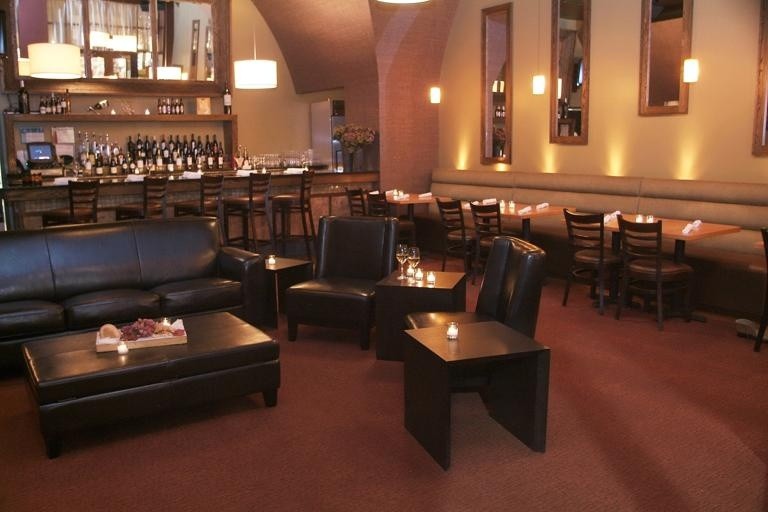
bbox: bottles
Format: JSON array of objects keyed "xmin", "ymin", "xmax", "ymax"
[
  {"xmin": 75, "ymin": 129, "xmax": 225, "ymax": 177},
  {"xmin": 232, "ymin": 144, "xmax": 248, "ymax": 170},
  {"xmin": 495, "ymin": 105, "xmax": 505, "ymax": 116},
  {"xmin": 250, "ymin": 152, "xmax": 306, "ymax": 169},
  {"xmin": 39, "ymin": 89, "xmax": 71, "ymax": 114},
  {"xmin": 157, "ymin": 98, "xmax": 184, "ymax": 114},
  {"xmin": 223, "ymin": 81, "xmax": 232, "ymax": 113},
  {"xmin": 557, "ymin": 96, "xmax": 568, "ymax": 121},
  {"xmin": 87, "ymin": 99, "xmax": 109, "ymax": 112},
  {"xmin": 17, "ymin": 78, "xmax": 30, "ymax": 114},
  {"xmin": 22, "ymin": 172, "xmax": 44, "ymax": 186}
]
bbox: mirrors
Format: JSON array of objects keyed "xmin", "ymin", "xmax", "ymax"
[
  {"xmin": 478, "ymin": 1, "xmax": 513, "ymax": 168},
  {"xmin": 635, "ymin": 0, "xmax": 696, "ymax": 119},
  {"xmin": 7, "ymin": 0, "xmax": 232, "ymax": 98},
  {"xmin": 548, "ymin": 0, "xmax": 591, "ymax": 149}
]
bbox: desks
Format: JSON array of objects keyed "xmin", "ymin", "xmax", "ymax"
[{"xmin": 393, "ymin": 314, "xmax": 560, "ymax": 474}]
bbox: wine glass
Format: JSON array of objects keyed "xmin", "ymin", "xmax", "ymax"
[{"xmin": 394, "ymin": 243, "xmax": 422, "ymax": 283}]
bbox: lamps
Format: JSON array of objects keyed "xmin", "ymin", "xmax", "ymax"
[
  {"xmin": 228, "ymin": 29, "xmax": 279, "ymax": 90},
  {"xmin": 681, "ymin": 57, "xmax": 700, "ymax": 84},
  {"xmin": 428, "ymin": 85, "xmax": 442, "ymax": 105},
  {"xmin": 16, "ymin": 28, "xmax": 87, "ymax": 82},
  {"xmin": 530, "ymin": 73, "xmax": 547, "ymax": 96}
]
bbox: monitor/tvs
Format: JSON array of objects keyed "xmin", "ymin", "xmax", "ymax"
[{"xmin": 27, "ymin": 142, "xmax": 55, "ymax": 163}]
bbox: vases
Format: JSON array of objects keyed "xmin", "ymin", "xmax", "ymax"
[{"xmin": 340, "ymin": 145, "xmax": 366, "ymax": 175}]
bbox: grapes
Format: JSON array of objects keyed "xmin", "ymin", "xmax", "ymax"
[{"xmin": 120, "ymin": 318, "xmax": 156, "ymax": 341}]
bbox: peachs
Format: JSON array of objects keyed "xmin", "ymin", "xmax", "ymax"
[{"xmin": 100, "ymin": 324, "xmax": 119, "ymax": 338}]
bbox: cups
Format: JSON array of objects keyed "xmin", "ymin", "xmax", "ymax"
[
  {"xmin": 413, "ymin": 267, "xmax": 424, "ymax": 280},
  {"xmin": 447, "ymin": 321, "xmax": 458, "ymax": 341},
  {"xmin": 509, "ymin": 200, "xmax": 516, "ymax": 209},
  {"xmin": 499, "ymin": 199, "xmax": 505, "ymax": 207},
  {"xmin": 392, "ymin": 189, "xmax": 403, "ymax": 197},
  {"xmin": 647, "ymin": 213, "xmax": 652, "ymax": 224},
  {"xmin": 162, "ymin": 317, "xmax": 171, "ymax": 325},
  {"xmin": 426, "ymin": 270, "xmax": 437, "ymax": 283},
  {"xmin": 636, "ymin": 214, "xmax": 642, "ymax": 223},
  {"xmin": 268, "ymin": 254, "xmax": 275, "ymax": 265},
  {"xmin": 117, "ymin": 339, "xmax": 129, "ymax": 353}
]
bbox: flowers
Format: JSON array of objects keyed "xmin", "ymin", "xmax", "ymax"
[{"xmin": 328, "ymin": 117, "xmax": 380, "ymax": 158}]
